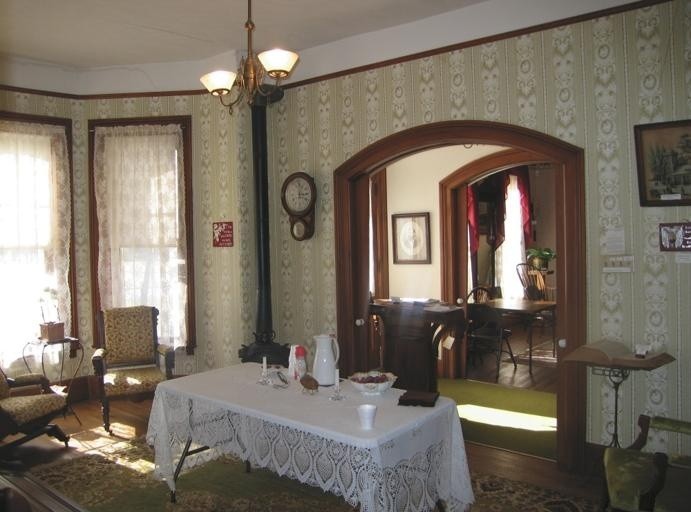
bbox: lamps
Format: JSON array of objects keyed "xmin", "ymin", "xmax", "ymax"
[{"xmin": 200, "ymin": 0, "xmax": 300, "ymax": 115}]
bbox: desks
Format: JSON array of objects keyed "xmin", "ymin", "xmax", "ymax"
[{"xmin": 356, "ymin": 299, "xmax": 463, "ymax": 380}]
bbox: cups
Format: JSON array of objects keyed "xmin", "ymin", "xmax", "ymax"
[{"xmin": 356, "ymin": 404, "xmax": 378, "ymax": 431}]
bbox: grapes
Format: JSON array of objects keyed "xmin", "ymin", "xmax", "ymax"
[{"xmin": 357, "ymin": 375, "xmax": 386, "ymax": 384}]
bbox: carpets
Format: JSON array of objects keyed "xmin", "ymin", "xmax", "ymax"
[{"xmin": 29, "ymin": 433, "xmax": 604, "ymax": 512}]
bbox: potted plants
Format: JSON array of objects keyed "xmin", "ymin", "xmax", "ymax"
[{"xmin": 526, "ymin": 246, "xmax": 556, "ymax": 271}]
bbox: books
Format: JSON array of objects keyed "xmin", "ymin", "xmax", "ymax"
[{"xmin": 561, "ymin": 336, "xmax": 679, "ymax": 369}]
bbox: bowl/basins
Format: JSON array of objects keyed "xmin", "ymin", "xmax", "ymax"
[{"xmin": 347, "ymin": 369, "xmax": 400, "ymax": 396}]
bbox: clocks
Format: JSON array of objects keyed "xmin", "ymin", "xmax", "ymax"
[{"xmin": 280, "ymin": 172, "xmax": 317, "ymax": 241}]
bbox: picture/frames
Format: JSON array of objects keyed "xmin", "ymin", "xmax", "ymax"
[
  {"xmin": 391, "ymin": 212, "xmax": 431, "ymax": 264},
  {"xmin": 634, "ymin": 118, "xmax": 691, "ymax": 252}
]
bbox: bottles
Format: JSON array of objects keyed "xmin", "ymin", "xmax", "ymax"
[{"xmin": 288, "ymin": 344, "xmax": 307, "ymax": 384}]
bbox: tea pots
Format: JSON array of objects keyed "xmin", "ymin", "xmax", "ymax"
[{"xmin": 312, "ymin": 332, "xmax": 342, "ymax": 386}]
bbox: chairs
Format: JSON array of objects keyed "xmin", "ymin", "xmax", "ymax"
[
  {"xmin": 0, "ymin": 305, "xmax": 174, "ymax": 465},
  {"xmin": 461, "ymin": 263, "xmax": 557, "ymax": 379}
]
